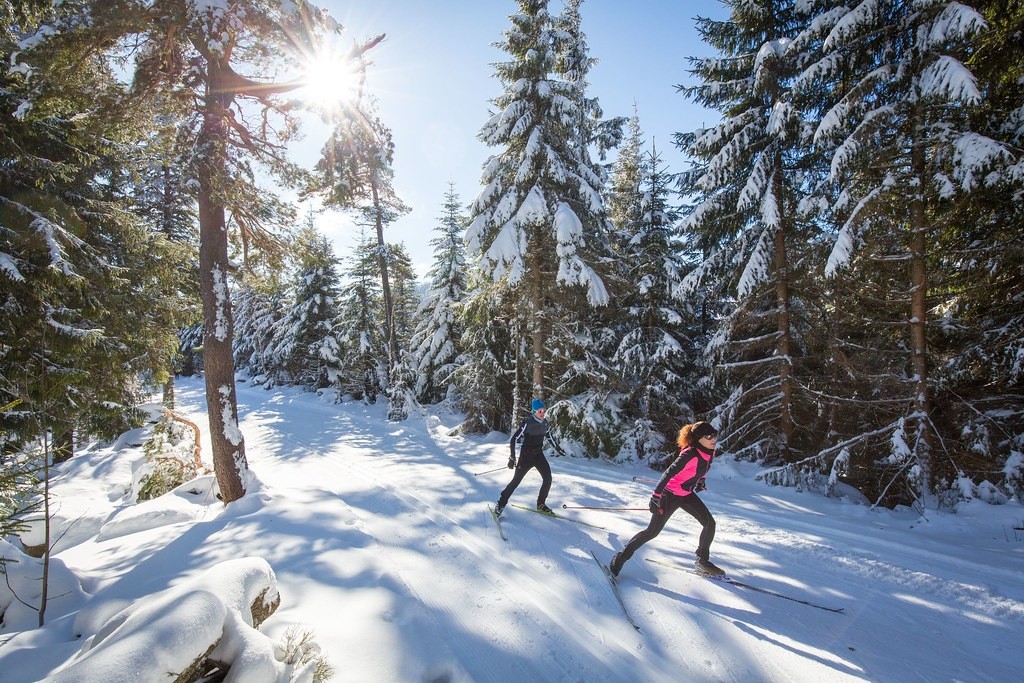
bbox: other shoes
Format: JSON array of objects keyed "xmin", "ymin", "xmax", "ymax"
[
  {"xmin": 610, "ymin": 552, "xmax": 623, "ymax": 576},
  {"xmin": 537, "ymin": 504, "xmax": 551, "ymax": 513},
  {"xmin": 697, "ymin": 559, "xmax": 725, "ymax": 576},
  {"xmin": 495, "ymin": 501, "xmax": 504, "ymax": 515}
]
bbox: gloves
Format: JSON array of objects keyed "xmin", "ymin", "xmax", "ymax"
[
  {"xmin": 556, "ymin": 448, "xmax": 565, "ymax": 455},
  {"xmin": 649, "ymin": 495, "xmax": 660, "ymax": 513},
  {"xmin": 508, "ymin": 459, "xmax": 515, "ymax": 469},
  {"xmin": 696, "ymin": 478, "xmax": 705, "ymax": 493}
]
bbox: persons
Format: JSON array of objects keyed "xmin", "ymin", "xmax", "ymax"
[
  {"xmin": 494, "ymin": 399, "xmax": 566, "ymax": 517},
  {"xmin": 610, "ymin": 421, "xmax": 725, "ymax": 579}
]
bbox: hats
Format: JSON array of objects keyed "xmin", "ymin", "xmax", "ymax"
[{"xmin": 532, "ymin": 400, "xmax": 544, "ymax": 414}]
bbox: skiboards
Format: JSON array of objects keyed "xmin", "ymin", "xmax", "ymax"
[
  {"xmin": 589, "ymin": 550, "xmax": 844, "ymax": 629},
  {"xmin": 487, "ymin": 504, "xmax": 605, "ymax": 542}
]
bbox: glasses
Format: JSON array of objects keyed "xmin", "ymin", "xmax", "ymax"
[
  {"xmin": 533, "ymin": 409, "xmax": 546, "ymax": 414},
  {"xmin": 703, "ymin": 430, "xmax": 717, "ymax": 440}
]
